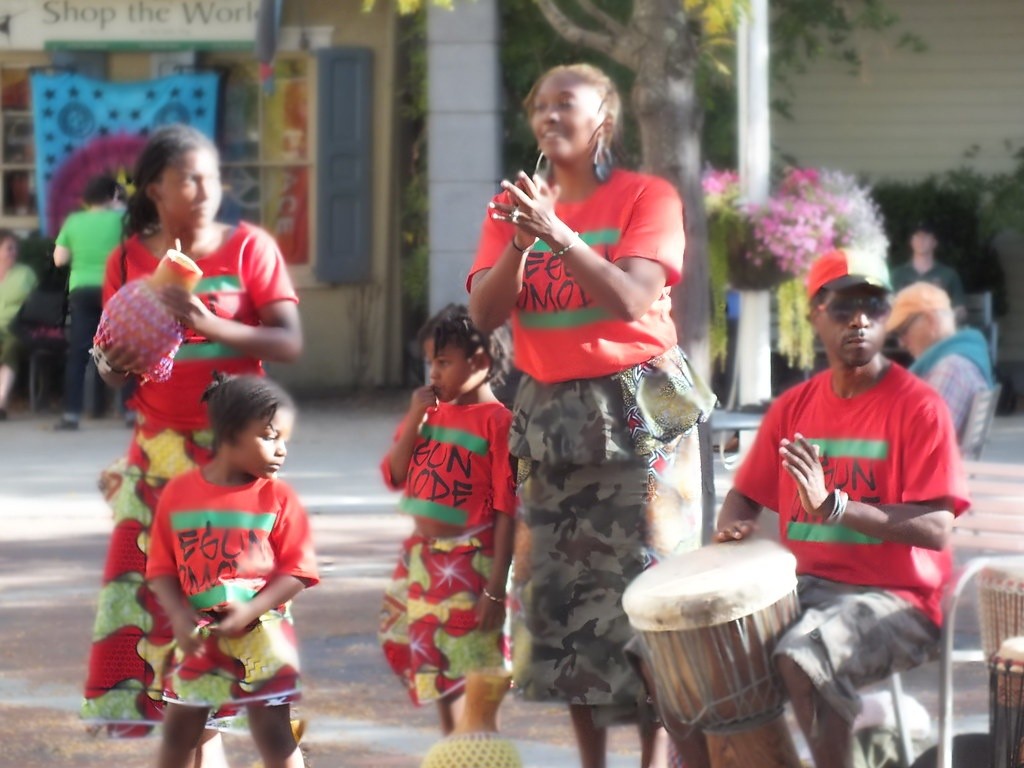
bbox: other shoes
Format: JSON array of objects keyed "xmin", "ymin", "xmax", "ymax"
[
  {"xmin": 53, "ymin": 417, "xmax": 76, "ymax": 428},
  {"xmin": 124, "ymin": 419, "xmax": 135, "ymax": 426}
]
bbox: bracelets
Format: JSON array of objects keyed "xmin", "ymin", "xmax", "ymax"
[
  {"xmin": 481, "ymin": 588, "xmax": 506, "ymax": 602},
  {"xmin": 548, "ymin": 231, "xmax": 579, "ymax": 256},
  {"xmin": 512, "ymin": 235, "xmax": 524, "ymax": 252},
  {"xmin": 822, "ymin": 486, "xmax": 850, "ymax": 526}
]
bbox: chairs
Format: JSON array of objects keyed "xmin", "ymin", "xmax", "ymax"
[
  {"xmin": 964, "ymin": 290, "xmax": 999, "ymax": 368},
  {"xmin": 7, "ymin": 289, "xmax": 69, "ymax": 411},
  {"xmin": 953, "ymin": 384, "xmax": 1004, "ymax": 451}
]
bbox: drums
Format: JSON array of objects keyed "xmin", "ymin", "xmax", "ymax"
[
  {"xmin": 618, "ymin": 541, "xmax": 805, "ymax": 768},
  {"xmin": 995, "ymin": 637, "xmax": 1023, "ymax": 768},
  {"xmin": 974, "ymin": 559, "xmax": 1024, "ymax": 768}
]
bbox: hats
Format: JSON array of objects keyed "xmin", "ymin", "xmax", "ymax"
[
  {"xmin": 809, "ymin": 249, "xmax": 890, "ymax": 305},
  {"xmin": 907, "ymin": 219, "xmax": 941, "ymax": 241}
]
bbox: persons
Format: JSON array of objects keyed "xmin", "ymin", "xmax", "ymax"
[
  {"xmin": 373, "ymin": 300, "xmax": 517, "ymax": 732},
  {"xmin": 884, "ymin": 282, "xmax": 994, "ymax": 445},
  {"xmin": 145, "ymin": 369, "xmax": 323, "ymax": 768},
  {"xmin": 650, "ymin": 246, "xmax": 972, "ymax": 767},
  {"xmin": 461, "ymin": 60, "xmax": 704, "ymax": 768},
  {"xmin": 72, "ymin": 122, "xmax": 303, "ymax": 768},
  {"xmin": 45, "ymin": 177, "xmax": 140, "ymax": 431},
  {"xmin": 0, "ymin": 227, "xmax": 41, "ymax": 422},
  {"xmin": 889, "ymin": 218, "xmax": 968, "ymax": 326}
]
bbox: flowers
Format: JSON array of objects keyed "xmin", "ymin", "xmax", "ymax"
[{"xmin": 702, "ymin": 162, "xmax": 902, "ymax": 370}]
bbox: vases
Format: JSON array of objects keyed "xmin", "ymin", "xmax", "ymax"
[{"xmin": 727, "ymin": 252, "xmax": 788, "ymax": 294}]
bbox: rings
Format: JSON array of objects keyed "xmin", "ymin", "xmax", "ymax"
[{"xmin": 512, "ymin": 207, "xmax": 521, "ymax": 219}]
